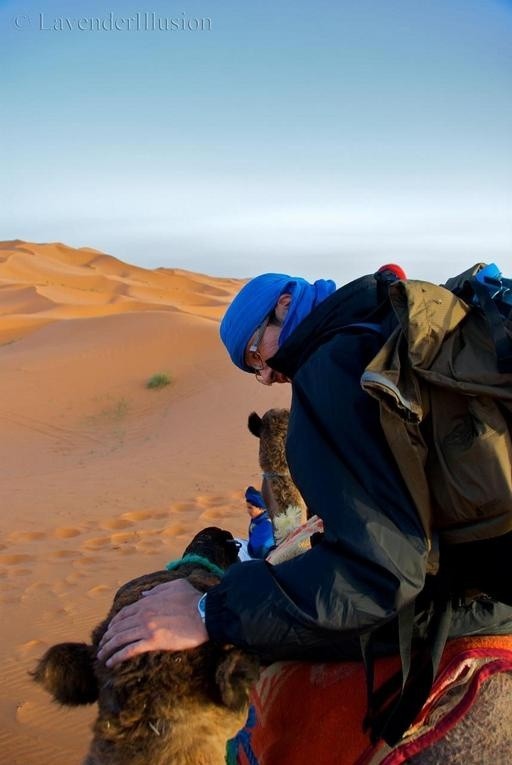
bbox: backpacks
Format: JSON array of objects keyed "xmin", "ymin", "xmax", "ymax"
[{"xmin": 360, "ymin": 260, "xmax": 511, "ymax": 578}]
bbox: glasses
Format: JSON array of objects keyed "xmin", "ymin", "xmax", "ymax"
[{"xmin": 247, "ymin": 314, "xmax": 272, "ymax": 372}]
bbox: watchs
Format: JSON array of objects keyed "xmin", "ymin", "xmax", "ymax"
[{"xmin": 197, "ymin": 590, "xmax": 208, "ymax": 623}]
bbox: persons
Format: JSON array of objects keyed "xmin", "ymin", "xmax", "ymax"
[
  {"xmin": 95, "ymin": 256, "xmax": 512, "ymax": 765},
  {"xmin": 241, "ymin": 485, "xmax": 280, "ymax": 565}
]
